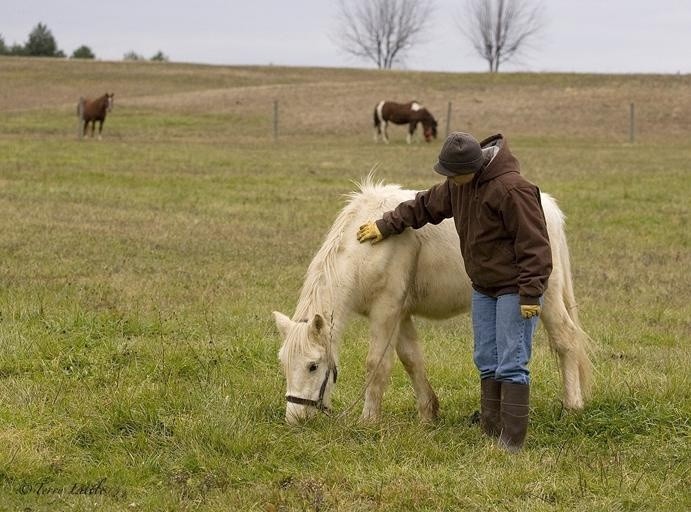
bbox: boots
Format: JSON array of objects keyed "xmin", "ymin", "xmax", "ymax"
[{"xmin": 480, "ymin": 379, "xmax": 530, "ymax": 446}]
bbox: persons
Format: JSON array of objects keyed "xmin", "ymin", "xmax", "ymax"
[{"xmin": 356, "ymin": 133, "xmax": 553, "ymax": 450}]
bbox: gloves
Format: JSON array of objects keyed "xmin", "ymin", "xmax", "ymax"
[
  {"xmin": 357, "ymin": 222, "xmax": 383, "ymax": 244},
  {"xmin": 521, "ymin": 304, "xmax": 540, "ymax": 319}
]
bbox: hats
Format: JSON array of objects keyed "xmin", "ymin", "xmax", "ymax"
[{"xmin": 434, "ymin": 132, "xmax": 485, "ymax": 176}]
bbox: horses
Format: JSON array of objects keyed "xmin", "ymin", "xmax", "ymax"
[
  {"xmin": 374, "ymin": 101, "xmax": 438, "ymax": 145},
  {"xmin": 273, "ymin": 162, "xmax": 605, "ymax": 426},
  {"xmin": 77, "ymin": 92, "xmax": 114, "ymax": 141}
]
